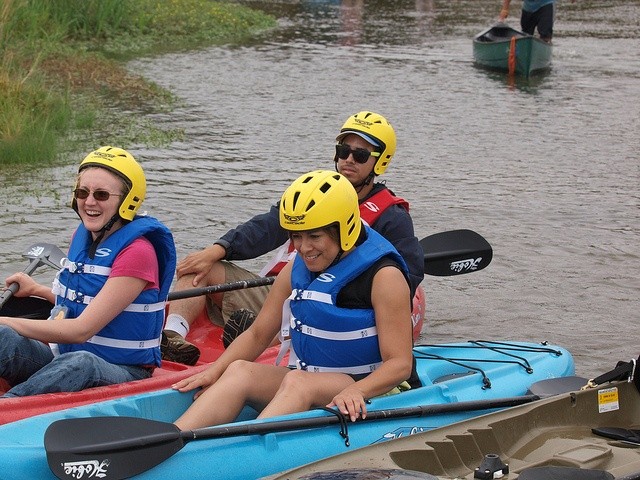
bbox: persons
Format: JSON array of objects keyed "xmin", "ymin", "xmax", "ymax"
[
  {"xmin": 0, "ymin": 146, "xmax": 175, "ymax": 400},
  {"xmin": 172, "ymin": 170, "xmax": 413, "ymax": 432},
  {"xmin": 161, "ymin": 111, "xmax": 425, "ymax": 365},
  {"xmin": 501, "ymin": 1, "xmax": 554, "ymax": 40}
]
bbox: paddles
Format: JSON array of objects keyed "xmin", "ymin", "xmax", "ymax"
[
  {"xmin": 42, "ymin": 374, "xmax": 592, "ymax": 479},
  {"xmin": 0, "ymin": 241, "xmax": 68, "ymax": 311},
  {"xmin": 164, "ymin": 228, "xmax": 493, "ymax": 303}
]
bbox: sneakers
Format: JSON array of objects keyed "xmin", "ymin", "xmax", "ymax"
[
  {"xmin": 158, "ymin": 329, "xmax": 199, "ymax": 366},
  {"xmin": 224, "ymin": 308, "xmax": 257, "ymax": 348}
]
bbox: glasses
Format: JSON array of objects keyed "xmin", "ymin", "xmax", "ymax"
[
  {"xmin": 73, "ymin": 188, "xmax": 124, "ymax": 202},
  {"xmin": 339, "ymin": 143, "xmax": 380, "ymax": 162}
]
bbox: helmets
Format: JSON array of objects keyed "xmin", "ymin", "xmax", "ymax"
[
  {"xmin": 277, "ymin": 170, "xmax": 361, "ymax": 273},
  {"xmin": 72, "ymin": 146, "xmax": 147, "ymax": 261},
  {"xmin": 333, "ymin": 110, "xmax": 397, "ymax": 193}
]
